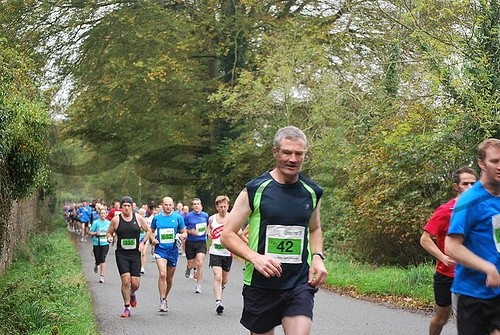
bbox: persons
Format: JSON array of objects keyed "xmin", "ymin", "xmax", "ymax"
[
  {"xmin": 183, "ymin": 198, "xmax": 209, "ymax": 293},
  {"xmin": 420, "ymin": 167, "xmax": 478, "ymax": 335},
  {"xmin": 107, "ymin": 196, "xmax": 151, "ymax": 318},
  {"xmin": 64, "ymin": 199, "xmax": 199, "ymax": 280},
  {"xmin": 221, "ymin": 126, "xmax": 328, "ymax": 335},
  {"xmin": 207, "ymin": 195, "xmax": 249, "ymax": 313},
  {"xmin": 444, "ymin": 139, "xmax": 500, "ymax": 335},
  {"xmin": 88, "ymin": 209, "xmax": 111, "ymax": 284},
  {"xmin": 149, "ymin": 197, "xmax": 188, "ymax": 311}
]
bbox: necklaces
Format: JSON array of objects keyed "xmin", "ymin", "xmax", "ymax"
[{"xmin": 142, "ymin": 239, "xmax": 146, "ymax": 245}]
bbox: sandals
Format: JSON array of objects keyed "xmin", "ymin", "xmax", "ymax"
[{"xmin": 94, "ymin": 264, "xmax": 100, "ymax": 273}]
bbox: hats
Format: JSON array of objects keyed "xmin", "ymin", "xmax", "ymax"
[{"xmin": 120, "ymin": 196, "xmax": 133, "ymax": 208}]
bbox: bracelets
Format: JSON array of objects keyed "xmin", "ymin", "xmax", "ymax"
[
  {"xmin": 95, "ymin": 232, "xmax": 97, "ymax": 235},
  {"xmin": 312, "ymin": 252, "xmax": 325, "ymax": 260}
]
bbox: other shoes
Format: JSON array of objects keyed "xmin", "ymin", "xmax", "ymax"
[
  {"xmin": 195, "ymin": 286, "xmax": 199, "ymax": 293},
  {"xmin": 99, "ymin": 275, "xmax": 104, "ymax": 283},
  {"xmin": 159, "ymin": 300, "xmax": 169, "ymax": 312},
  {"xmin": 130, "ymin": 295, "xmax": 136, "ymax": 307},
  {"xmin": 216, "ymin": 301, "xmax": 224, "ymax": 314},
  {"xmin": 121, "ymin": 308, "xmax": 130, "ymax": 317},
  {"xmin": 72, "ymin": 228, "xmax": 89, "ymax": 242},
  {"xmin": 185, "ymin": 269, "xmax": 191, "ymax": 278}
]
